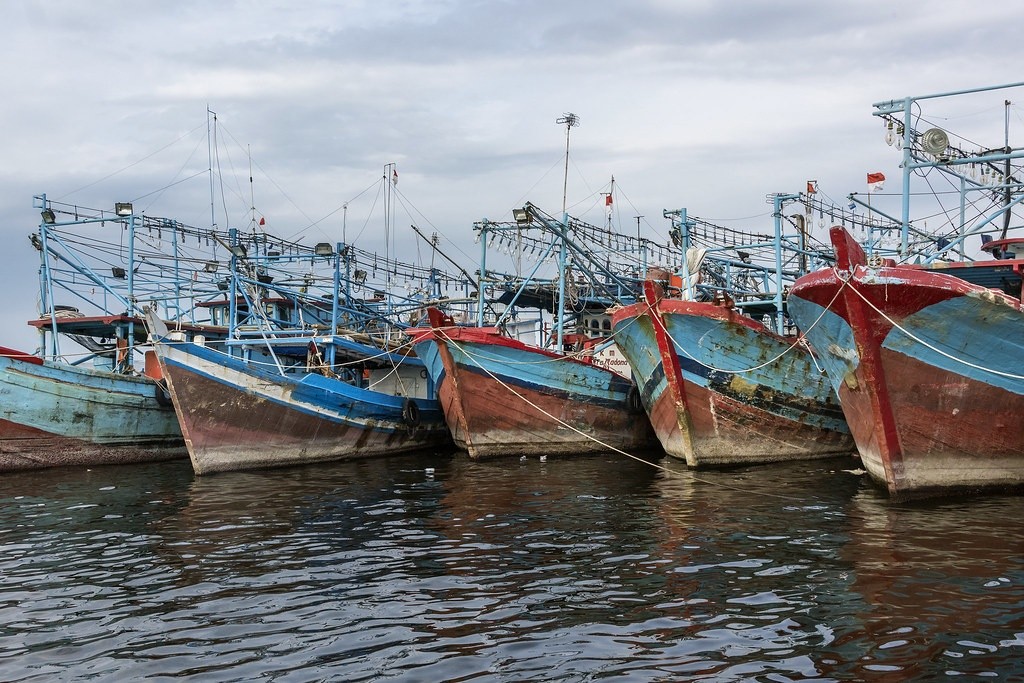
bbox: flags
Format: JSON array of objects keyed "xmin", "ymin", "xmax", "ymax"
[
  {"xmin": 808, "ymin": 183, "xmax": 817, "ymax": 194},
  {"xmin": 259, "ymin": 218, "xmax": 265, "ymax": 225},
  {"xmin": 868, "ymin": 173, "xmax": 885, "ymax": 184},
  {"xmin": 606, "ymin": 196, "xmax": 612, "ymax": 206}
]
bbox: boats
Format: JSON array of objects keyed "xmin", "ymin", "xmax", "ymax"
[{"xmin": 0, "ymin": 80, "xmax": 1024, "ymax": 503}]
